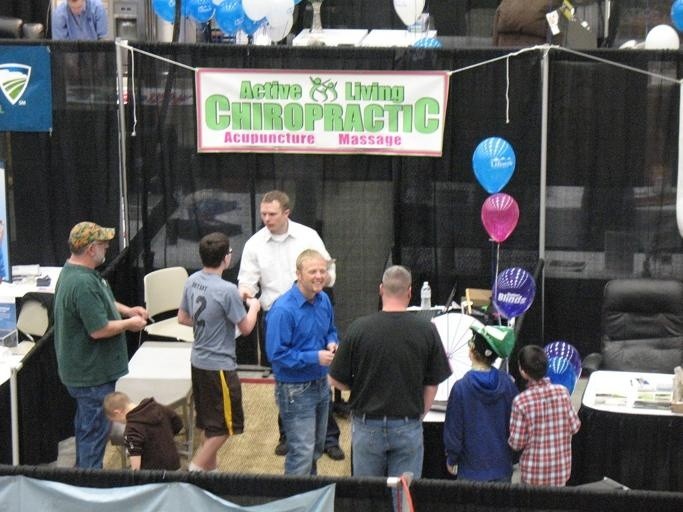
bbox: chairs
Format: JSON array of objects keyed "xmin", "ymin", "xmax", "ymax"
[
  {"xmin": 138, "ymin": 265, "xmax": 191, "ymax": 346},
  {"xmin": 582, "ymin": 277, "xmax": 683, "ymax": 373}
]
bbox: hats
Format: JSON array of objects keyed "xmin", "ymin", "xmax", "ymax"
[
  {"xmin": 469, "ymin": 325, "xmax": 516, "ymax": 361},
  {"xmin": 68, "ymin": 222, "xmax": 116, "ymax": 253}
]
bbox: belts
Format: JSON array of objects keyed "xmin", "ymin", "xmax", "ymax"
[{"xmin": 353, "ymin": 408, "xmax": 417, "ymax": 420}]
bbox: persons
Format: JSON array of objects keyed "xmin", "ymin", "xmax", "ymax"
[
  {"xmin": 53, "ymin": 221, "xmax": 151, "ymax": 470},
  {"xmin": 103, "ymin": 390, "xmax": 184, "ymax": 470},
  {"xmin": 327, "ymin": 265, "xmax": 453, "ymax": 480},
  {"xmin": 490, "ymin": 0, "xmax": 565, "ymax": 46},
  {"xmin": 265, "ymin": 246, "xmax": 343, "ymax": 478},
  {"xmin": 176, "ymin": 230, "xmax": 263, "ymax": 475},
  {"xmin": 234, "ymin": 189, "xmax": 347, "ymax": 462},
  {"xmin": 504, "ymin": 344, "xmax": 582, "ymax": 486},
  {"xmin": 441, "ymin": 323, "xmax": 521, "ymax": 482},
  {"xmin": 51, "ymin": 0, "xmax": 111, "ymax": 39}
]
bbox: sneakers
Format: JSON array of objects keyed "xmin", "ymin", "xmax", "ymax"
[
  {"xmin": 276, "ymin": 441, "xmax": 288, "ymax": 454},
  {"xmin": 325, "ymin": 445, "xmax": 344, "ymax": 459}
]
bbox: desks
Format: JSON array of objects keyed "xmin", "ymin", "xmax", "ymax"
[
  {"xmin": 579, "ymin": 370, "xmax": 682, "ymax": 495},
  {"xmin": 115, "ymin": 339, "xmax": 202, "ymax": 461}
]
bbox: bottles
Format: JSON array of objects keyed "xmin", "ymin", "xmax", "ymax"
[{"xmin": 418, "ymin": 280, "xmax": 433, "ymax": 308}]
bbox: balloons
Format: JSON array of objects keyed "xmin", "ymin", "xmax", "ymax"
[
  {"xmin": 645, "ymin": 0, "xmax": 682, "ymax": 50},
  {"xmin": 480, "ymin": 192, "xmax": 521, "ymax": 242},
  {"xmin": 546, "ymin": 355, "xmax": 578, "ymax": 396},
  {"xmin": 542, "ymin": 339, "xmax": 584, "ymax": 380},
  {"xmin": 491, "ymin": 267, "xmax": 537, "ymax": 319},
  {"xmin": 471, "ymin": 136, "xmax": 516, "ymax": 194},
  {"xmin": 389, "ymin": 0, "xmax": 442, "ymax": 48},
  {"xmin": 150, "ymin": 0, "xmax": 302, "ymax": 43}
]
